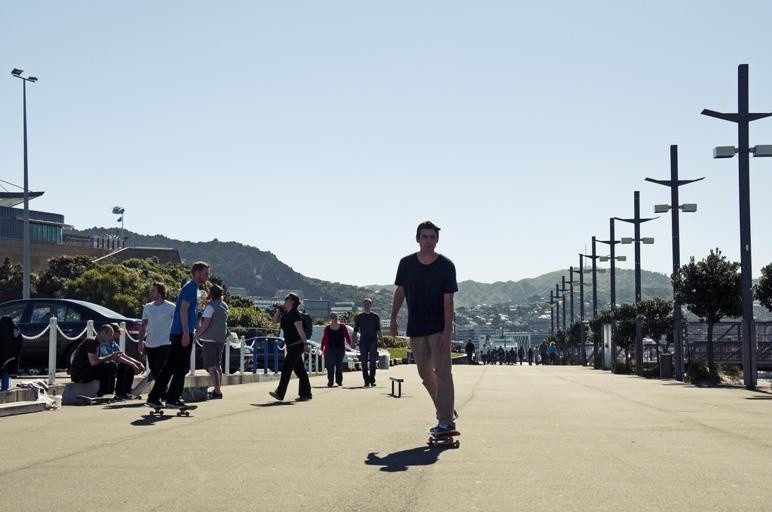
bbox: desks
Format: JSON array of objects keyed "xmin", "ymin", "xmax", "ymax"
[
  {"xmin": 546, "ymin": 189, "xmax": 660, "ymax": 375},
  {"xmin": 644, "ymin": 145, "xmax": 705, "ymax": 382},
  {"xmin": 700, "ymin": 63, "xmax": 771, "ymax": 387},
  {"xmin": 11, "ymin": 68, "xmax": 38, "ymax": 300}
]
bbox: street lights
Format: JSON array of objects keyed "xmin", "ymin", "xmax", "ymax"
[{"xmin": 300, "ymin": 313, "xmax": 312, "ymax": 339}]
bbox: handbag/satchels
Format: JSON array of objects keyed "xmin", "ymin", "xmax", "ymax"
[{"xmin": 303, "ymin": 343, "xmax": 308, "ymax": 346}]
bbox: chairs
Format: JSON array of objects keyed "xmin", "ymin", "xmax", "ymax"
[
  {"xmin": 328, "ymin": 380, "xmax": 342, "ymax": 386},
  {"xmin": 166, "ymin": 401, "xmax": 186, "ymax": 408},
  {"xmin": 453, "ymin": 411, "xmax": 458, "ymax": 419},
  {"xmin": 296, "ymin": 394, "xmax": 313, "ymax": 401},
  {"xmin": 270, "ymin": 392, "xmax": 281, "ymax": 400},
  {"xmin": 147, "ymin": 399, "xmax": 163, "ymax": 407},
  {"xmin": 430, "ymin": 424, "xmax": 455, "ymax": 433},
  {"xmin": 364, "ymin": 383, "xmax": 376, "ymax": 387},
  {"xmin": 209, "ymin": 390, "xmax": 223, "ymax": 399}
]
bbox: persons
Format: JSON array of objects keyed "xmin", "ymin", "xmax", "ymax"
[
  {"xmin": 72, "ymin": 324, "xmax": 124, "ymax": 402},
  {"xmin": 463, "ymin": 339, "xmax": 558, "ymax": 366},
  {"xmin": 351, "ymin": 297, "xmax": 388, "ymax": 389},
  {"xmin": 390, "ymin": 223, "xmax": 459, "ymax": 435},
  {"xmin": 138, "ymin": 281, "xmax": 176, "ymax": 404},
  {"xmin": 198, "ymin": 281, "xmax": 230, "ymax": 397},
  {"xmin": 99, "ymin": 323, "xmax": 146, "ymax": 402},
  {"xmin": 147, "ymin": 261, "xmax": 211, "ymax": 408},
  {"xmin": 271, "ymin": 291, "xmax": 314, "ymax": 401},
  {"xmin": 319, "ymin": 313, "xmax": 356, "ymax": 388}
]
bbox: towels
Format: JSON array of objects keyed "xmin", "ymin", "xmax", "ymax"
[{"xmin": 659, "ymin": 354, "xmax": 673, "ymax": 379}]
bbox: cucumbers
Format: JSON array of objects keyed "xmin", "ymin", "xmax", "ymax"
[
  {"xmin": 429, "ymin": 429, "xmax": 461, "ymax": 447},
  {"xmin": 129, "ymin": 371, "xmax": 153, "ymax": 399},
  {"xmin": 144, "ymin": 404, "xmax": 197, "ymax": 417},
  {"xmin": 75, "ymin": 393, "xmax": 117, "ymax": 406}
]
